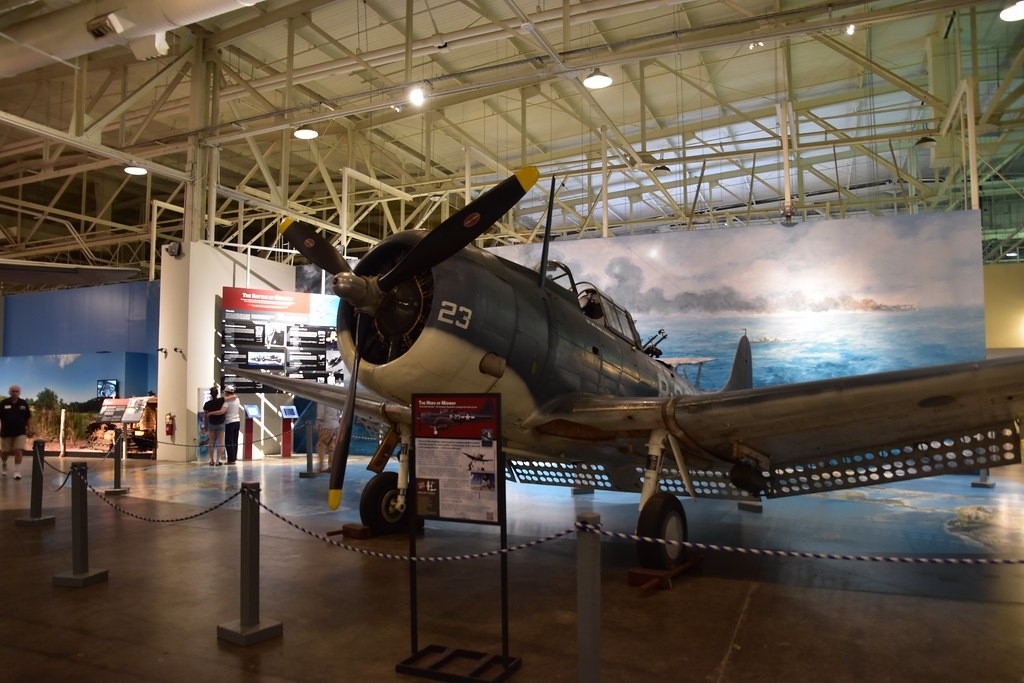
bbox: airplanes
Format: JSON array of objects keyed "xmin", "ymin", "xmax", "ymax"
[{"xmin": 221, "ymin": 163, "xmax": 1024, "ymax": 569}]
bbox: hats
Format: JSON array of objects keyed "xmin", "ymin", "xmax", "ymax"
[
  {"xmin": 210, "ymin": 387, "xmax": 218, "ymax": 394},
  {"xmin": 224, "ymin": 385, "xmax": 235, "ymax": 393}
]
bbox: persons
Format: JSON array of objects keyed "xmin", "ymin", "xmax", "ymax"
[
  {"xmin": 0, "ymin": 385, "xmax": 33, "ymax": 480},
  {"xmin": 202, "ymin": 385, "xmax": 240, "ymax": 466},
  {"xmin": 335, "ymin": 377, "xmax": 344, "ymax": 387},
  {"xmin": 316, "ymin": 402, "xmax": 340, "ymax": 472}
]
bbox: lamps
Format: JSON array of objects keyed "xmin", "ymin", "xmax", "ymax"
[
  {"xmin": 583, "ymin": 68, "xmax": 613, "ymax": 89},
  {"xmin": 124, "ymin": 167, "xmax": 147, "ymax": 176},
  {"xmin": 1000, "ymin": 0, "xmax": 1024, "ymax": 23},
  {"xmin": 293, "ymin": 123, "xmax": 320, "ymax": 139}
]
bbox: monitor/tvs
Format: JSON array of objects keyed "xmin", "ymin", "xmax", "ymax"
[{"xmin": 96, "ymin": 379, "xmax": 118, "ymax": 398}]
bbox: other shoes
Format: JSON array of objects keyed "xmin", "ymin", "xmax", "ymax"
[
  {"xmin": 3, "ymin": 463, "xmax": 7, "ymax": 475},
  {"xmin": 225, "ymin": 460, "xmax": 236, "ymax": 464},
  {"xmin": 324, "ymin": 466, "xmax": 332, "ymax": 472},
  {"xmin": 209, "ymin": 459, "xmax": 214, "ymax": 466},
  {"xmin": 216, "ymin": 461, "xmax": 222, "ymax": 466},
  {"xmin": 14, "ymin": 472, "xmax": 21, "ymax": 479}
]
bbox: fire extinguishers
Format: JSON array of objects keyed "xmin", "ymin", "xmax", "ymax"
[{"xmin": 166, "ymin": 413, "xmax": 176, "ymax": 436}]
